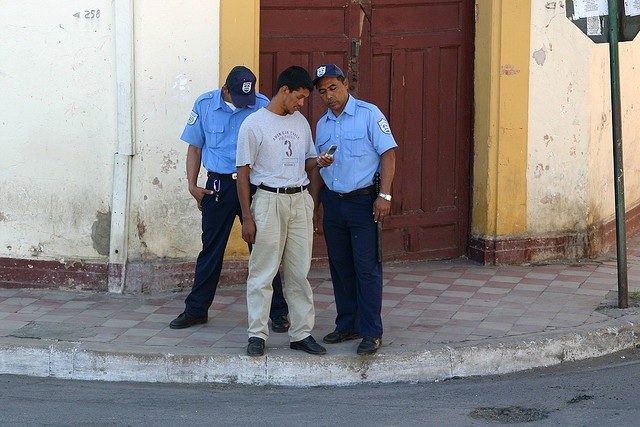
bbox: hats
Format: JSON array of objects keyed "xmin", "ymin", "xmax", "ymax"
[
  {"xmin": 226, "ymin": 65, "xmax": 256, "ymax": 106},
  {"xmin": 311, "ymin": 63, "xmax": 346, "ymax": 84}
]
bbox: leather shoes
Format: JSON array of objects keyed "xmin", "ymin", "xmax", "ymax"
[
  {"xmin": 248, "ymin": 335, "xmax": 265, "ymax": 355},
  {"xmin": 290, "ymin": 335, "xmax": 327, "ymax": 354},
  {"xmin": 323, "ymin": 330, "xmax": 362, "ymax": 343},
  {"xmin": 357, "ymin": 335, "xmax": 382, "ymax": 353},
  {"xmin": 272, "ymin": 314, "xmax": 289, "ymax": 331},
  {"xmin": 170, "ymin": 310, "xmax": 208, "ymax": 329}
]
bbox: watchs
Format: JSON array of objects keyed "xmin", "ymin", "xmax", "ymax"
[{"xmin": 378, "ymin": 192, "xmax": 391, "ymax": 200}]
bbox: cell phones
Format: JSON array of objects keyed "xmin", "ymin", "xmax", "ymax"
[{"xmin": 325, "ymin": 144, "xmax": 337, "ymax": 157}]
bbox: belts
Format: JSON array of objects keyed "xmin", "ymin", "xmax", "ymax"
[
  {"xmin": 205, "ymin": 170, "xmax": 238, "ymax": 179},
  {"xmin": 324, "ymin": 186, "xmax": 377, "ymax": 196},
  {"xmin": 258, "ymin": 182, "xmax": 309, "ymax": 195}
]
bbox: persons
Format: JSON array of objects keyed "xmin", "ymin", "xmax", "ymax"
[
  {"xmin": 235, "ymin": 66, "xmax": 337, "ymax": 355},
  {"xmin": 170, "ymin": 65, "xmax": 289, "ymax": 332},
  {"xmin": 308, "ymin": 64, "xmax": 399, "ymax": 352}
]
